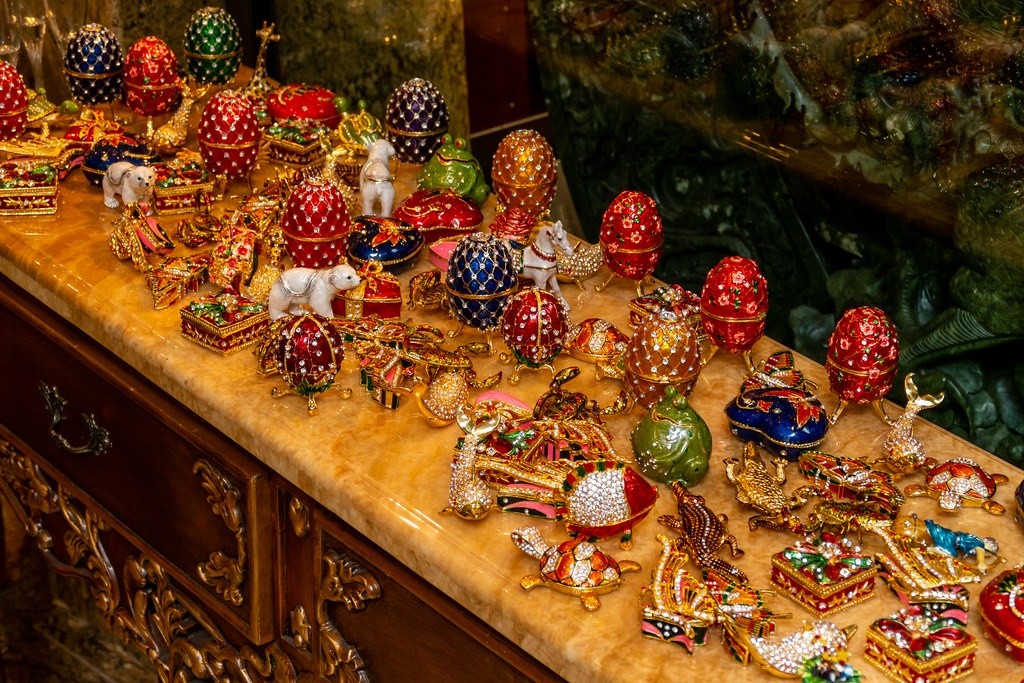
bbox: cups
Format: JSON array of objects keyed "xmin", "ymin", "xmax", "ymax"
[{"xmin": 0, "ymin": 0, "xmax": 21, "ymax": 74}]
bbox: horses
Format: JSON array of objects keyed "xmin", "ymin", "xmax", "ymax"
[{"xmin": 502, "ymin": 219, "xmax": 574, "ymax": 310}]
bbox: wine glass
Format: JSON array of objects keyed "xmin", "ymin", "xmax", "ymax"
[
  {"xmin": 6, "ymin": 0, "xmax": 48, "ymax": 98},
  {"xmin": 43, "ymin": 0, "xmax": 88, "ymax": 58}
]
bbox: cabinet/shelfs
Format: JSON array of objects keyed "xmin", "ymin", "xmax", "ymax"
[{"xmin": 0, "ymin": 64, "xmax": 1024, "ymax": 683}]
